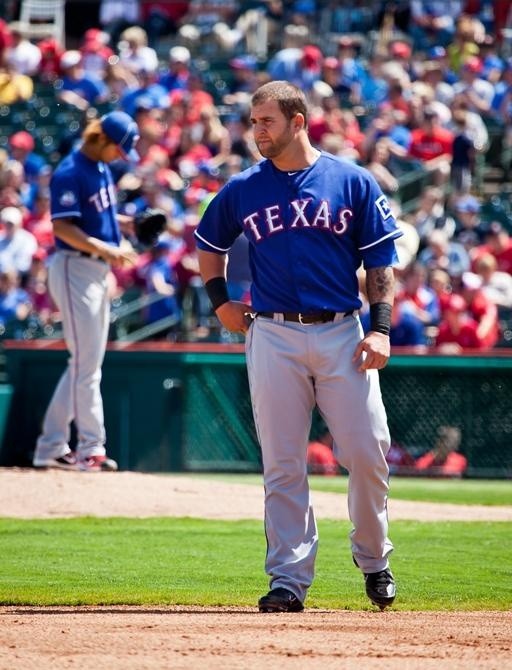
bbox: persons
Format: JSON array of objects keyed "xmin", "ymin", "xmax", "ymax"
[
  {"xmin": 415, "ymin": 423, "xmax": 466, "ymax": 477},
  {"xmin": 306, "ymin": 424, "xmax": 342, "ymax": 477},
  {"xmin": 192, "ymin": 81, "xmax": 401, "ymax": 612},
  {"xmin": 383, "ymin": 441, "xmax": 414, "ymax": 476},
  {"xmin": 1, "ymin": 0, "xmax": 512, "ymax": 355},
  {"xmin": 31, "ymin": 111, "xmax": 142, "ymax": 472}
]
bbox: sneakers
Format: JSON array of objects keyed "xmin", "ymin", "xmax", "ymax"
[
  {"xmin": 352, "ymin": 555, "xmax": 396, "ymax": 607},
  {"xmin": 259, "ymin": 587, "xmax": 304, "ymax": 612},
  {"xmin": 75, "ymin": 450, "xmax": 118, "ymax": 472},
  {"xmin": 33, "ymin": 451, "xmax": 83, "ymax": 470}
]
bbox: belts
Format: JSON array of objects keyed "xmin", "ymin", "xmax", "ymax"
[
  {"xmin": 257, "ymin": 311, "xmax": 353, "ymax": 326},
  {"xmin": 80, "ymin": 251, "xmax": 103, "ymax": 260}
]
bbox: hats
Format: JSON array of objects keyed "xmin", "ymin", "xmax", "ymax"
[{"xmin": 100, "ymin": 110, "xmax": 140, "ymax": 161}]
